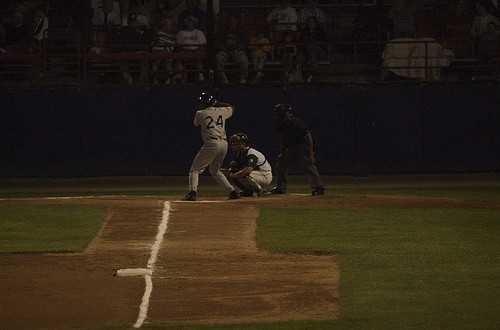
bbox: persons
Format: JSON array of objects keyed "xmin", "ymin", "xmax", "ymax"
[
  {"xmin": 272, "ymin": 102, "xmax": 325, "ymax": 195},
  {"xmin": 227, "ymin": 133, "xmax": 274, "ymax": 197},
  {"xmin": 0, "ymin": 0, "xmax": 500, "ymax": 81},
  {"xmin": 184, "ymin": 90, "xmax": 239, "ymax": 200}
]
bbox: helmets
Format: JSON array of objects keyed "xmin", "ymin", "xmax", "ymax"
[
  {"xmin": 195, "ymin": 89, "xmax": 216, "ymax": 106},
  {"xmin": 274, "ymin": 103, "xmax": 291, "ymax": 113},
  {"xmin": 229, "ymin": 133, "xmax": 248, "ymax": 142}
]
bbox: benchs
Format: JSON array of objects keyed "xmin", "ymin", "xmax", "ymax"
[{"xmin": 0, "ymin": 5, "xmax": 500, "ymax": 82}]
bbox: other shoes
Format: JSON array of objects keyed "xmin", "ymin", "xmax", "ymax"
[
  {"xmin": 312, "ymin": 187, "xmax": 324, "ymax": 196},
  {"xmin": 228, "ymin": 189, "xmax": 240, "ymax": 199},
  {"xmin": 183, "ymin": 190, "xmax": 197, "ymax": 201},
  {"xmin": 239, "ymin": 191, "xmax": 255, "ymax": 197},
  {"xmin": 270, "ymin": 187, "xmax": 287, "ymax": 194}
]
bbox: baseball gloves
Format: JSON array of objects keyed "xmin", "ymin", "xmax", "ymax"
[{"xmin": 223, "ymin": 160, "xmax": 238, "ymax": 170}]
